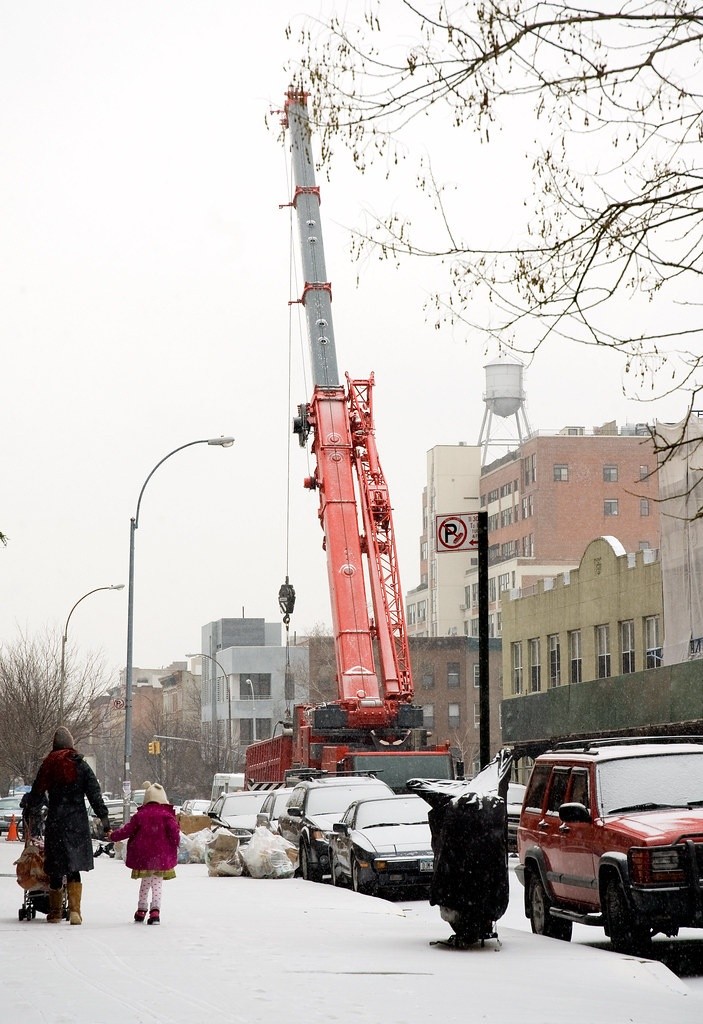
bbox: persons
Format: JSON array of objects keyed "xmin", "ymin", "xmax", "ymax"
[
  {"xmin": 19, "ymin": 781, "xmax": 49, "ymax": 840},
  {"xmin": 107, "ymin": 783, "xmax": 181, "ymax": 925},
  {"xmin": 29, "ymin": 726, "xmax": 112, "ymax": 925}
]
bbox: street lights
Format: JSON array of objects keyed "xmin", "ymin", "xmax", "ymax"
[
  {"xmin": 58, "ymin": 583, "xmax": 126, "ymax": 727},
  {"xmin": 245, "ymin": 679, "xmax": 256, "ymax": 743},
  {"xmin": 123, "ymin": 436, "xmax": 237, "ymax": 827},
  {"xmin": 185, "ymin": 653, "xmax": 231, "ymax": 773}
]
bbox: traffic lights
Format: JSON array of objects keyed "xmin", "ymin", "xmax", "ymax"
[
  {"xmin": 148, "ymin": 742, "xmax": 154, "ymax": 755},
  {"xmin": 155, "ymin": 741, "xmax": 161, "ymax": 755}
]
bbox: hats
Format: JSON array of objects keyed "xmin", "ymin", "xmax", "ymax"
[
  {"xmin": 140, "ymin": 781, "xmax": 169, "ymax": 804},
  {"xmin": 52, "ymin": 726, "xmax": 73, "ymax": 749}
]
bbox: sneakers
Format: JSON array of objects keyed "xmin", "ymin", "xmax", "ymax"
[
  {"xmin": 147, "ymin": 911, "xmax": 160, "ymax": 925},
  {"xmin": 134, "ymin": 911, "xmax": 145, "ymax": 923}
]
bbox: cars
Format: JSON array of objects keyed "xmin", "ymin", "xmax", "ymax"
[
  {"xmin": 0, "ymin": 790, "xmax": 146, "ymax": 841},
  {"xmin": 507, "ymin": 782, "xmax": 528, "ymax": 852},
  {"xmin": 180, "ymin": 800, "xmax": 194, "ymax": 815},
  {"xmin": 256, "ymin": 786, "xmax": 294, "ymax": 834},
  {"xmin": 328, "ymin": 794, "xmax": 433, "ymax": 895},
  {"xmin": 188, "ymin": 799, "xmax": 212, "ymax": 815},
  {"xmin": 206, "ymin": 790, "xmax": 272, "ymax": 845}
]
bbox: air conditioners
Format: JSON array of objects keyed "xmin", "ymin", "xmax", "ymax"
[
  {"xmin": 460, "ymin": 604, "xmax": 467, "ymax": 609},
  {"xmin": 472, "ymin": 601, "xmax": 475, "ymax": 607}
]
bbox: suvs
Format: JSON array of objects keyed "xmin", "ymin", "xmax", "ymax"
[
  {"xmin": 276, "ymin": 775, "xmax": 397, "ymax": 882},
  {"xmin": 514, "ymin": 735, "xmax": 703, "ymax": 960}
]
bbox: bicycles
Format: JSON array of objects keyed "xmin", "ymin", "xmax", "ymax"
[{"xmin": 16, "ymin": 809, "xmax": 46, "ymax": 846}]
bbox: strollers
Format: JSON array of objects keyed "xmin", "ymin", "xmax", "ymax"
[{"xmin": 13, "ymin": 844, "xmax": 71, "ymax": 923}]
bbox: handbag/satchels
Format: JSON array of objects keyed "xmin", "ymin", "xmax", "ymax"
[{"xmin": 13, "ymin": 845, "xmax": 48, "ymax": 891}]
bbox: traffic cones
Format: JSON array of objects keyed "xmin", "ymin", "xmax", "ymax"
[{"xmin": 5, "ymin": 814, "xmax": 18, "ymax": 841}]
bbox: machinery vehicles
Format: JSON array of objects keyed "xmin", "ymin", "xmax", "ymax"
[{"xmin": 244, "ymin": 89, "xmax": 467, "ymax": 791}]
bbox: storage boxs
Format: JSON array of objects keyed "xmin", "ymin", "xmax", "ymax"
[
  {"xmin": 208, "ymin": 850, "xmax": 235, "ymax": 877},
  {"xmin": 208, "ymin": 834, "xmax": 238, "ymax": 852},
  {"xmin": 285, "ymin": 848, "xmax": 298, "ymax": 866}
]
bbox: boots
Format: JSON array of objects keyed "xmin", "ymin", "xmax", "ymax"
[
  {"xmin": 67, "ymin": 882, "xmax": 82, "ymax": 924},
  {"xmin": 46, "ymin": 884, "xmax": 62, "ymax": 922}
]
